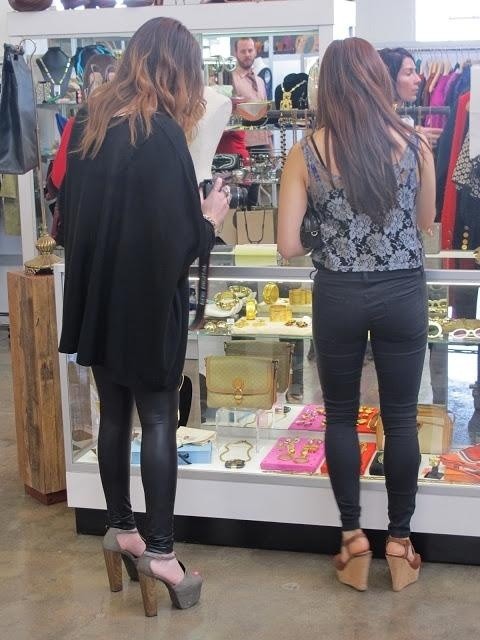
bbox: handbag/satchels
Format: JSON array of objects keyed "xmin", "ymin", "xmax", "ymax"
[
  {"xmin": 369, "ymin": 404, "xmax": 453, "ymax": 455},
  {"xmin": 224, "ymin": 340, "xmax": 295, "ymax": 393},
  {"xmin": 0, "ymin": 43, "xmax": 40, "ymax": 175},
  {"xmin": 198, "ymin": 153, "xmax": 243, "ymax": 208},
  {"xmin": 204, "ymin": 355, "xmax": 279, "ymax": 410},
  {"xmin": 300, "ymin": 209, "xmax": 323, "ymax": 251}
]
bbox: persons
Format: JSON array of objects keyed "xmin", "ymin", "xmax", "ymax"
[
  {"xmin": 378, "ymin": 47, "xmax": 440, "ymax": 147},
  {"xmin": 229, "ymin": 38, "xmax": 271, "ymax": 206},
  {"xmin": 278, "ymin": 36, "xmax": 437, "ymax": 592},
  {"xmin": 55, "ymin": 16, "xmax": 232, "ymax": 618}
]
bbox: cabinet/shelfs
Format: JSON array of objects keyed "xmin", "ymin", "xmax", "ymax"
[
  {"xmin": 9, "ymin": 0, "xmax": 356, "ymax": 265},
  {"xmin": 5, "ymin": 263, "xmax": 68, "ymax": 506}
]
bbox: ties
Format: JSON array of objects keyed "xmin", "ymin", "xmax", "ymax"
[{"xmin": 246, "ymin": 72, "xmax": 258, "ymax": 92}]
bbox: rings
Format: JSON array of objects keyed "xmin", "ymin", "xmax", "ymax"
[
  {"xmin": 226, "ymin": 197, "xmax": 231, "ymax": 205},
  {"xmin": 220, "ymin": 188, "xmax": 229, "ymax": 195}
]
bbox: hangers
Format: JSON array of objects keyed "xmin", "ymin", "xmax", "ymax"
[{"xmin": 408, "ymin": 47, "xmax": 480, "ymax": 99}]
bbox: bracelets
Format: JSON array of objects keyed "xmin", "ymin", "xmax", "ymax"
[
  {"xmin": 204, "ymin": 282, "xmax": 314, "ymax": 334},
  {"xmin": 204, "ymin": 214, "xmax": 217, "ymax": 236}
]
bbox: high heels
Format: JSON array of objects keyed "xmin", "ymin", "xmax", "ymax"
[
  {"xmin": 384, "ymin": 536, "xmax": 422, "ymax": 592},
  {"xmin": 136, "ymin": 549, "xmax": 203, "ymax": 617},
  {"xmin": 332, "ymin": 532, "xmax": 373, "ymax": 591},
  {"xmin": 102, "ymin": 527, "xmax": 147, "ymax": 593}
]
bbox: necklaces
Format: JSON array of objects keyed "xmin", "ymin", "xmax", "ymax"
[
  {"xmin": 39, "ymin": 56, "xmax": 72, "ymax": 97},
  {"xmin": 281, "ymin": 80, "xmax": 306, "ymax": 111},
  {"xmin": 242, "ymin": 210, "xmax": 267, "ymax": 246}
]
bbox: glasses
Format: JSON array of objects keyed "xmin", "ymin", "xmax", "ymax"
[
  {"xmin": 448, "ymin": 328, "xmax": 480, "ymax": 339},
  {"xmin": 427, "ymin": 320, "xmax": 444, "ymax": 339}
]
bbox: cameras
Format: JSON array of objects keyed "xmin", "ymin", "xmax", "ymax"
[{"xmin": 199, "ymin": 179, "xmax": 249, "ymax": 209}]
